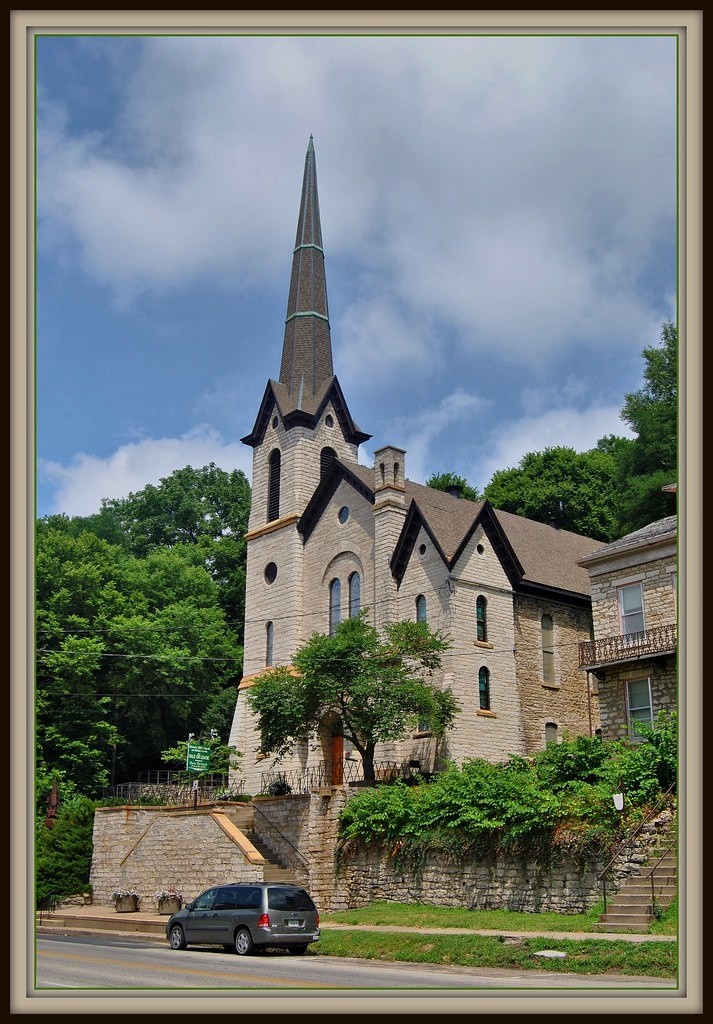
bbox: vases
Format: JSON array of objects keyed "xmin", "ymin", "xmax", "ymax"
[
  {"xmin": 116, "ymin": 896, "xmax": 137, "ymax": 913},
  {"xmin": 158, "ymin": 899, "xmax": 181, "ymax": 915}
]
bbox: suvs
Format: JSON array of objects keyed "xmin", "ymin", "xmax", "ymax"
[{"xmin": 166, "ymin": 879, "xmax": 320, "ymax": 957}]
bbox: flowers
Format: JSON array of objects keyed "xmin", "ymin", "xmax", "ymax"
[
  {"xmin": 111, "ymin": 887, "xmax": 141, "ymax": 902},
  {"xmin": 153, "ymin": 890, "xmax": 183, "ymax": 901}
]
